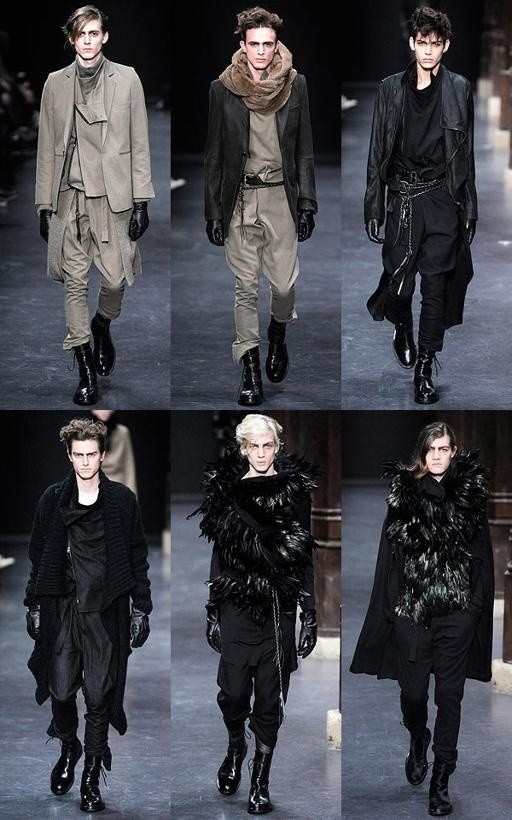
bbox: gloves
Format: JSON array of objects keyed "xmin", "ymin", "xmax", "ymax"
[
  {"xmin": 297, "ymin": 212, "xmax": 315, "ymax": 241},
  {"xmin": 206, "ymin": 220, "xmax": 224, "ymax": 246},
  {"xmin": 24, "ymin": 605, "xmax": 39, "ymax": 640},
  {"xmin": 366, "ymin": 218, "xmax": 386, "ymax": 245},
  {"xmin": 129, "ymin": 607, "xmax": 150, "ymax": 647},
  {"xmin": 204, "ymin": 602, "xmax": 221, "ymax": 653},
  {"xmin": 39, "ymin": 209, "xmax": 51, "ymax": 240},
  {"xmin": 129, "ymin": 203, "xmax": 149, "ymax": 240},
  {"xmin": 465, "ymin": 219, "xmax": 477, "ymax": 243},
  {"xmin": 297, "ymin": 610, "xmax": 318, "ymax": 659}
]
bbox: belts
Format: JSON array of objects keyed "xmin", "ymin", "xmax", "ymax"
[
  {"xmin": 239, "ymin": 173, "xmax": 286, "ymax": 190},
  {"xmin": 387, "ymin": 181, "xmax": 446, "ymax": 196}
]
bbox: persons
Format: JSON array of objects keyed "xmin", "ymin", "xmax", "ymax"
[
  {"xmin": 35, "ymin": 5, "xmax": 156, "ymax": 406},
  {"xmin": 364, "ymin": 5, "xmax": 478, "ymax": 404},
  {"xmin": 35, "ymin": 71, "xmax": 40, "ymax": 138},
  {"xmin": 185, "ymin": 414, "xmax": 318, "ymax": 814},
  {"xmin": 348, "ymin": 421, "xmax": 495, "ymax": 816},
  {"xmin": 0, "ymin": 71, "xmax": 35, "ymax": 208},
  {"xmin": 204, "ymin": 6, "xmax": 319, "ymax": 406},
  {"xmin": 22, "ymin": 416, "xmax": 153, "ymax": 813},
  {"xmin": 90, "ymin": 410, "xmax": 140, "ymax": 505}
]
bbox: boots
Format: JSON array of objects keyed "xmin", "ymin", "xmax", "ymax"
[
  {"xmin": 414, "ymin": 347, "xmax": 439, "ymax": 404},
  {"xmin": 430, "ymin": 762, "xmax": 452, "ymax": 816},
  {"xmin": 393, "ymin": 321, "xmax": 417, "ymax": 369},
  {"xmin": 216, "ymin": 727, "xmax": 248, "ymax": 794},
  {"xmin": 267, "ymin": 315, "xmax": 289, "ymax": 383},
  {"xmin": 50, "ymin": 736, "xmax": 83, "ymax": 796},
  {"xmin": 406, "ymin": 722, "xmax": 431, "ymax": 785},
  {"xmin": 80, "ymin": 756, "xmax": 106, "ymax": 811},
  {"xmin": 248, "ymin": 749, "xmax": 272, "ymax": 814},
  {"xmin": 238, "ymin": 347, "xmax": 263, "ymax": 404},
  {"xmin": 74, "ymin": 343, "xmax": 97, "ymax": 405},
  {"xmin": 90, "ymin": 310, "xmax": 114, "ymax": 376}
]
